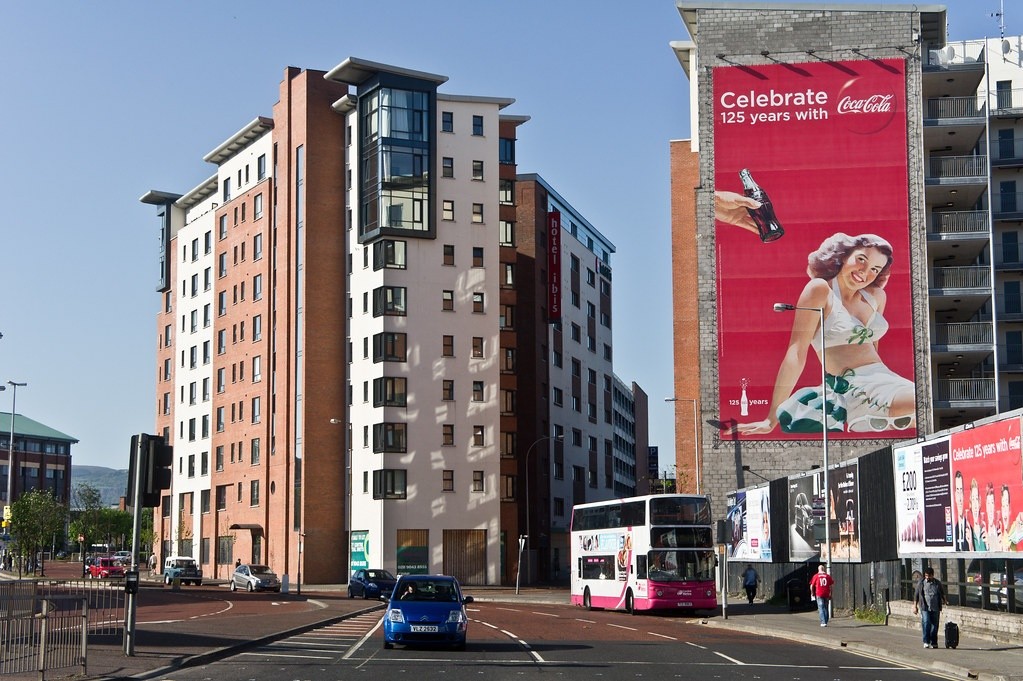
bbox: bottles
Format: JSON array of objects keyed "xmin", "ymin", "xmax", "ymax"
[{"xmin": 738, "ymin": 168, "xmax": 785, "ymax": 242}]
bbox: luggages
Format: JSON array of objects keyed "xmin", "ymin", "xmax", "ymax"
[{"xmin": 944, "ymin": 602, "xmax": 959, "ymax": 649}]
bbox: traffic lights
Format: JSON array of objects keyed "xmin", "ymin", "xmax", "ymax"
[{"xmin": 147, "ymin": 439, "xmax": 173, "ymax": 494}]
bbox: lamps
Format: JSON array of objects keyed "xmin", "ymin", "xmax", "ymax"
[
  {"xmin": 895, "ymin": 45, "xmax": 920, "ymax": 61},
  {"xmin": 742, "ymin": 466, "xmax": 770, "ymax": 482},
  {"xmin": 761, "ymin": 51, "xmax": 779, "ymax": 63},
  {"xmin": 805, "ymin": 50, "xmax": 824, "ymax": 61},
  {"xmin": 716, "ymin": 54, "xmax": 736, "ymax": 64},
  {"xmin": 850, "ymin": 48, "xmax": 870, "ymax": 59}
]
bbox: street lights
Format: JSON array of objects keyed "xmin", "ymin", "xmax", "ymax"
[
  {"xmin": 2, "ymin": 380, "xmax": 28, "ymax": 572},
  {"xmin": 526, "ymin": 434, "xmax": 565, "ymax": 585},
  {"xmin": 773, "ymin": 302, "xmax": 834, "ymax": 618},
  {"xmin": 664, "ymin": 397, "xmax": 700, "ymax": 495}
]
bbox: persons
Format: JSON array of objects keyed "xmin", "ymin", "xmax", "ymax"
[
  {"xmin": 761, "ymin": 494, "xmax": 771, "ymax": 557},
  {"xmin": 401, "ymin": 582, "xmax": 418, "ymax": 599},
  {"xmin": 3, "ymin": 553, "xmax": 12, "ymax": 571},
  {"xmin": 236, "ymin": 559, "xmax": 241, "ymax": 568},
  {"xmin": 741, "ymin": 564, "xmax": 762, "ymax": 607},
  {"xmin": 715, "ymin": 191, "xmax": 916, "ymax": 435},
  {"xmin": 913, "ymin": 568, "xmax": 949, "ymax": 649},
  {"xmin": 955, "ymin": 471, "xmax": 1016, "ymax": 549},
  {"xmin": 809, "ymin": 565, "xmax": 834, "ymax": 627},
  {"xmin": 150, "ymin": 553, "xmax": 157, "ymax": 576}
]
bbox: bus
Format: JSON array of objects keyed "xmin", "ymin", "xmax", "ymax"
[{"xmin": 569, "ymin": 493, "xmax": 719, "ymax": 617}]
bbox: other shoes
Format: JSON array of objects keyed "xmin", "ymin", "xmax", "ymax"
[
  {"xmin": 820, "ymin": 623, "xmax": 827, "ymax": 627},
  {"xmin": 749, "ymin": 603, "xmax": 753, "ymax": 605},
  {"xmin": 930, "ymin": 644, "xmax": 938, "ymax": 648},
  {"xmin": 923, "ymin": 643, "xmax": 929, "ymax": 648}
]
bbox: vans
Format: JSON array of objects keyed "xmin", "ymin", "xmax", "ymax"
[{"xmin": 965, "ymin": 558, "xmax": 1023, "ymax": 608}]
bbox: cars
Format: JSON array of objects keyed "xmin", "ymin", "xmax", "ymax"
[
  {"xmin": 230, "ymin": 564, "xmax": 282, "ymax": 593},
  {"xmin": 977, "ymin": 567, "xmax": 1023, "ymax": 614},
  {"xmin": 164, "ymin": 557, "xmax": 203, "ymax": 586},
  {"xmin": 84, "ymin": 551, "xmax": 132, "ymax": 579},
  {"xmin": 380, "ymin": 574, "xmax": 474, "ymax": 651},
  {"xmin": 347, "ymin": 569, "xmax": 398, "ymax": 601}
]
